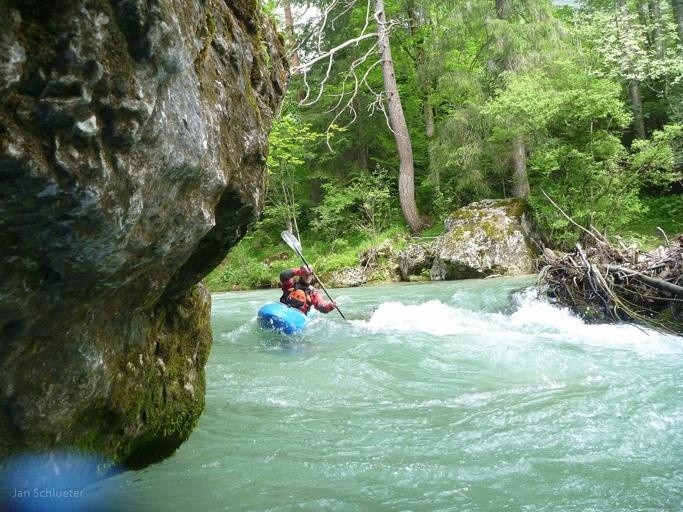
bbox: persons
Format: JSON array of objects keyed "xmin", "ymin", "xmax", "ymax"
[{"xmin": 280, "ymin": 265, "xmax": 339, "ymax": 316}]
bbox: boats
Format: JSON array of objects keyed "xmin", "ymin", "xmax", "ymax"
[{"xmin": 252, "ymin": 298, "xmax": 323, "ymax": 345}]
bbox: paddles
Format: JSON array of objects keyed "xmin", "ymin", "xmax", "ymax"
[{"xmin": 280, "ymin": 231, "xmax": 348, "ymax": 321}]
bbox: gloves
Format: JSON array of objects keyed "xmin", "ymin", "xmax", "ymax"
[
  {"xmin": 302, "ymin": 265, "xmax": 313, "ymax": 275},
  {"xmin": 331, "ymin": 300, "xmax": 340, "ymax": 309}
]
